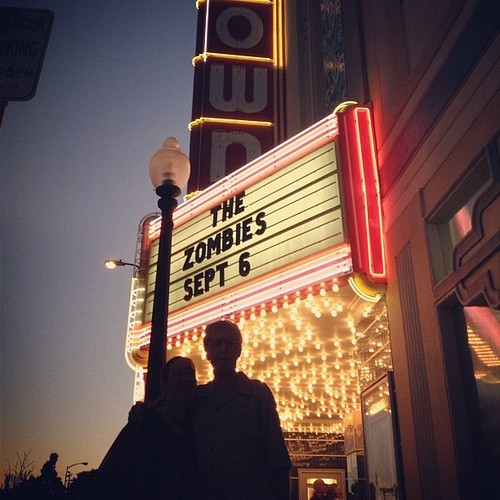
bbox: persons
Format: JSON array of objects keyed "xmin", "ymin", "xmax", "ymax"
[
  {"xmin": 309, "ymin": 480, "xmax": 338, "ymax": 500},
  {"xmin": 99, "ymin": 356, "xmax": 201, "ymax": 500},
  {"xmin": 189, "ymin": 320, "xmax": 293, "ymax": 500}
]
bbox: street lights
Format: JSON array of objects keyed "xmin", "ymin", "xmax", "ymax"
[{"xmin": 142, "ymin": 135, "xmax": 191, "ymax": 402}]
